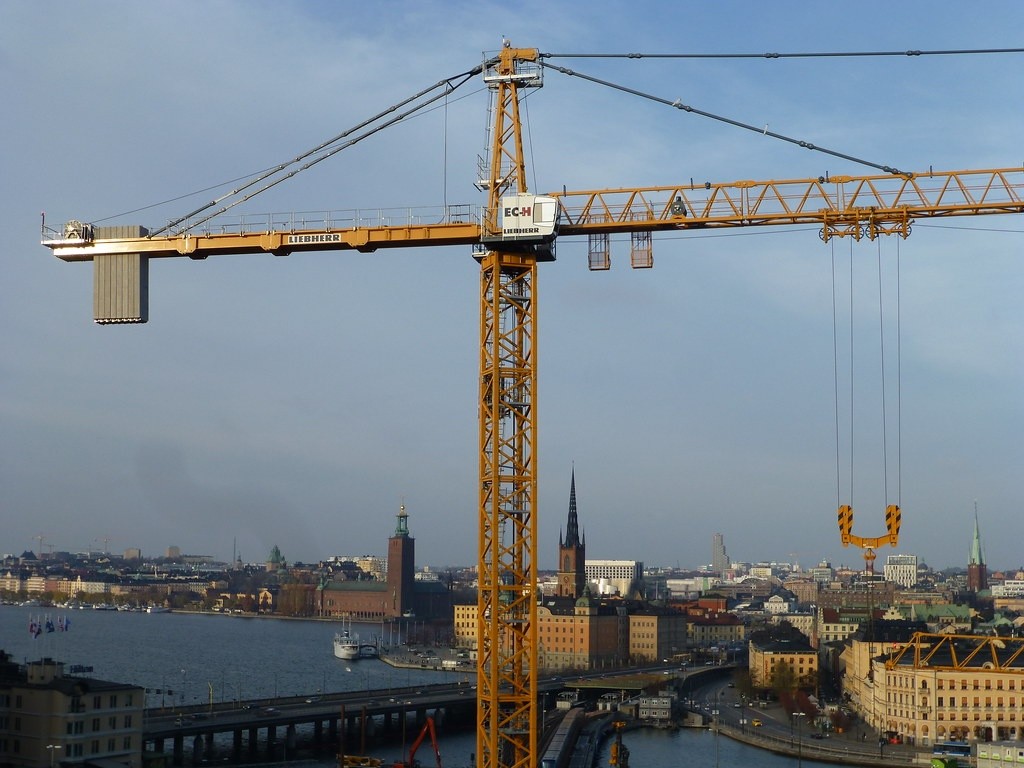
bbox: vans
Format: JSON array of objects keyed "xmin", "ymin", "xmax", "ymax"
[{"xmin": 305, "ymin": 697, "xmax": 320, "ymax": 703}]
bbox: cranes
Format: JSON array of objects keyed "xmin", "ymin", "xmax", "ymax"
[{"xmin": 41, "ymin": 40, "xmax": 1024, "ymax": 768}]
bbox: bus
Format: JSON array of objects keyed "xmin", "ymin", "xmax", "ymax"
[{"xmin": 933, "ymin": 743, "xmax": 971, "ymax": 757}]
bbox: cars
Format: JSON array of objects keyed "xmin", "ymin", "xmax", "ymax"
[
  {"xmin": 263, "ymin": 707, "xmax": 281, "ymax": 716},
  {"xmin": 426, "ymin": 656, "xmax": 438, "ymax": 660},
  {"xmin": 408, "ymin": 647, "xmax": 417, "ymax": 651},
  {"xmin": 578, "ymin": 659, "xmax": 724, "ymax": 682},
  {"xmin": 417, "ymin": 650, "xmax": 437, "ymax": 658},
  {"xmin": 174, "ymin": 718, "xmax": 192, "ymax": 726},
  {"xmin": 734, "ymin": 702, "xmax": 741, "ymax": 709},
  {"xmin": 811, "ymin": 732, "xmax": 823, "ymax": 739},
  {"xmin": 242, "ymin": 703, "xmax": 258, "ymax": 711},
  {"xmin": 388, "ymin": 696, "xmax": 401, "ymax": 702},
  {"xmin": 192, "ymin": 714, "xmax": 209, "ymax": 720},
  {"xmin": 752, "ymin": 719, "xmax": 763, "ymax": 727},
  {"xmin": 403, "ymin": 684, "xmax": 478, "ymax": 704},
  {"xmin": 551, "ymin": 676, "xmax": 563, "ymax": 681}
]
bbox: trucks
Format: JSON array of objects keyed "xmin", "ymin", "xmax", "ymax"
[{"xmin": 457, "ymin": 654, "xmax": 469, "ymax": 658}]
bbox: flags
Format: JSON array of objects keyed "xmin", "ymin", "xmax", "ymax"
[
  {"xmin": 64, "ymin": 618, "xmax": 71, "ymax": 631},
  {"xmin": 34, "ymin": 622, "xmax": 42, "ymax": 640},
  {"xmin": 50, "ymin": 619, "xmax": 55, "ymax": 632},
  {"xmin": 57, "ymin": 619, "xmax": 65, "ymax": 632},
  {"xmin": 29, "ymin": 619, "xmax": 33, "ymax": 634},
  {"xmin": 45, "ymin": 618, "xmax": 51, "ymax": 633}
]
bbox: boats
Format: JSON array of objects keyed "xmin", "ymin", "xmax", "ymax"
[
  {"xmin": 1, "ymin": 598, "xmax": 170, "ymax": 613},
  {"xmin": 334, "ymin": 630, "xmax": 361, "ymax": 661}
]
